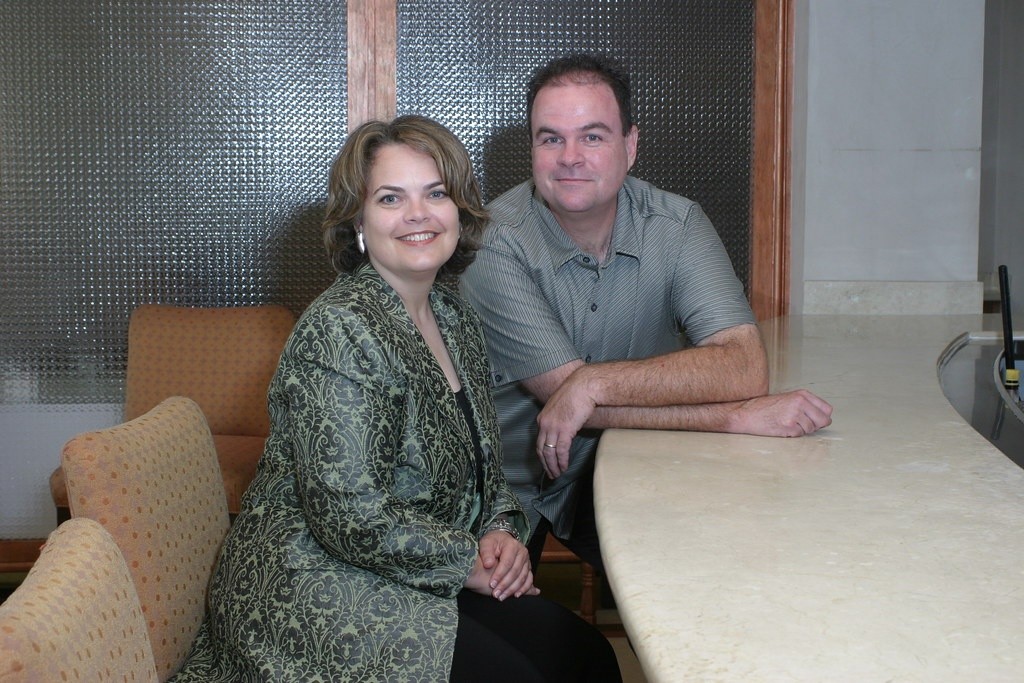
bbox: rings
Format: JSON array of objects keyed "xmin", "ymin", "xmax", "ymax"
[{"xmin": 544, "ymin": 444, "xmax": 556, "ymax": 448}]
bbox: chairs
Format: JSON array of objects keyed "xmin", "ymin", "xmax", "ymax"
[
  {"xmin": 60, "ymin": 394, "xmax": 229, "ymax": 683},
  {"xmin": 49, "ymin": 303, "xmax": 297, "ymax": 527},
  {"xmin": 0, "ymin": 516, "xmax": 160, "ymax": 683}
]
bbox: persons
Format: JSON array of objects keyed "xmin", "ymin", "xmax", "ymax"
[
  {"xmin": 175, "ymin": 116, "xmax": 622, "ymax": 682},
  {"xmin": 457, "ymin": 53, "xmax": 833, "ymax": 584}
]
